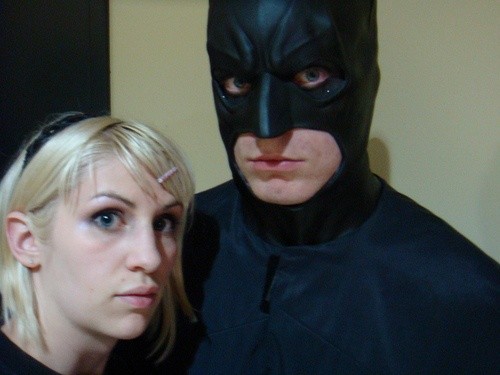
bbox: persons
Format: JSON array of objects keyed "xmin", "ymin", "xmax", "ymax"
[
  {"xmin": 103, "ymin": 0, "xmax": 500, "ymax": 375},
  {"xmin": 0, "ymin": 112, "xmax": 196, "ymax": 375}
]
